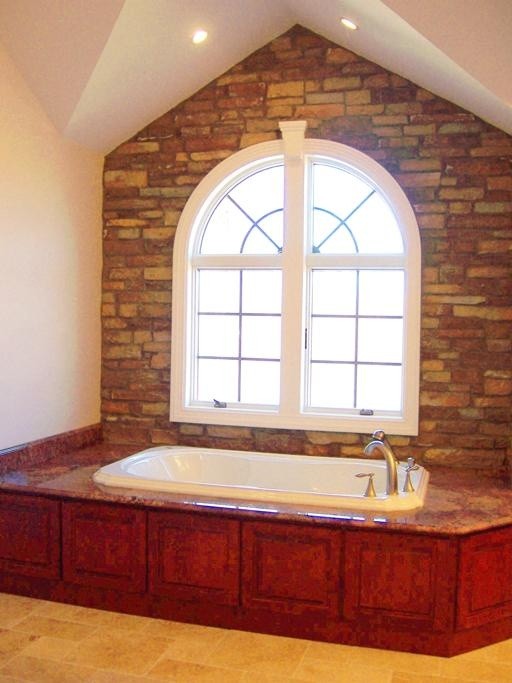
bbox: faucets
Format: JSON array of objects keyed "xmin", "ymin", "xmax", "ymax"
[{"xmin": 362, "ymin": 439, "xmax": 401, "ymax": 496}]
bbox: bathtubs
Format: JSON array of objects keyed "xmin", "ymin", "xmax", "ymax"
[{"xmin": 91, "ymin": 443, "xmax": 431, "ymax": 514}]
